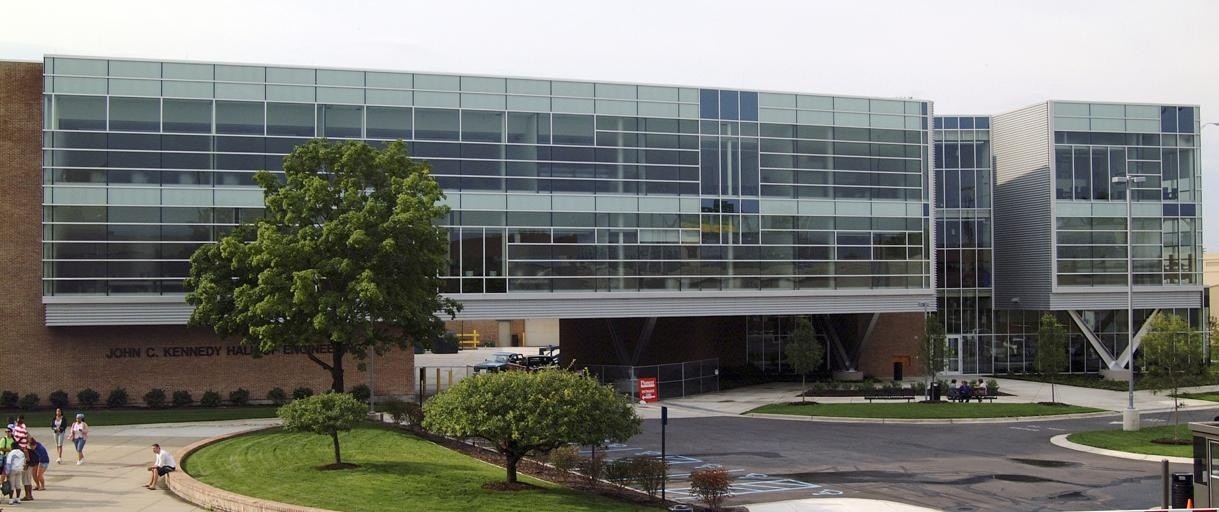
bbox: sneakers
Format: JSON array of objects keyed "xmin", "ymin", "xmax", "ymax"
[
  {"xmin": 9, "ymin": 498, "xmax": 15, "ymax": 505},
  {"xmin": 13, "ymin": 498, "xmax": 20, "ymax": 503},
  {"xmin": 56, "ymin": 458, "xmax": 86, "ymax": 465}
]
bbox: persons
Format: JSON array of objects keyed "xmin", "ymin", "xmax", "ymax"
[
  {"xmin": 0, "ymin": 415, "xmax": 50, "ymax": 504},
  {"xmin": 67, "ymin": 414, "xmax": 89, "ymax": 465},
  {"xmin": 959, "ymin": 381, "xmax": 972, "ymax": 402},
  {"xmin": 50, "ymin": 408, "xmax": 67, "ymax": 464},
  {"xmin": 948, "ymin": 379, "xmax": 960, "ymax": 403},
  {"xmin": 975, "ymin": 379, "xmax": 988, "ymax": 402},
  {"xmin": 142, "ymin": 444, "xmax": 175, "ymax": 490}
]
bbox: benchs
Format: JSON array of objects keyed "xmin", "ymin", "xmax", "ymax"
[
  {"xmin": 948, "ymin": 393, "xmax": 997, "ymax": 404},
  {"xmin": 864, "ymin": 394, "xmax": 916, "ymax": 404}
]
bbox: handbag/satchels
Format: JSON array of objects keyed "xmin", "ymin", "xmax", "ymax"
[
  {"xmin": 0, "ymin": 453, "xmax": 6, "ymax": 474},
  {"xmin": 1, "ymin": 481, "xmax": 12, "ymax": 495}
]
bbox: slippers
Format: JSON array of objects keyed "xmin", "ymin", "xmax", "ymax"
[{"xmin": 21, "ymin": 487, "xmax": 46, "ymax": 502}]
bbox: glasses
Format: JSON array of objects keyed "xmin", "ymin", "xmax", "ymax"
[{"xmin": 5, "ymin": 431, "xmax": 11, "ymax": 433}]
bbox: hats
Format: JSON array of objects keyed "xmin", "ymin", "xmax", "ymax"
[{"xmin": 18, "ymin": 414, "xmax": 24, "ymax": 421}]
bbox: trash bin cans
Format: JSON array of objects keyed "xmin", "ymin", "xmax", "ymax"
[
  {"xmin": 929, "ymin": 381, "xmax": 941, "ymax": 400},
  {"xmin": 1170, "ymin": 472, "xmax": 1195, "ymax": 509}
]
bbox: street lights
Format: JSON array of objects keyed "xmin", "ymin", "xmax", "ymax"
[
  {"xmin": 919, "ymin": 301, "xmax": 930, "ymax": 401},
  {"xmin": 1113, "ymin": 174, "xmax": 1146, "ymax": 431},
  {"xmin": 1012, "ymin": 298, "xmax": 1025, "ymax": 377}
]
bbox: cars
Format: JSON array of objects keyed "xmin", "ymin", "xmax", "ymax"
[{"xmin": 472, "ymin": 352, "xmax": 561, "ymax": 375}]
